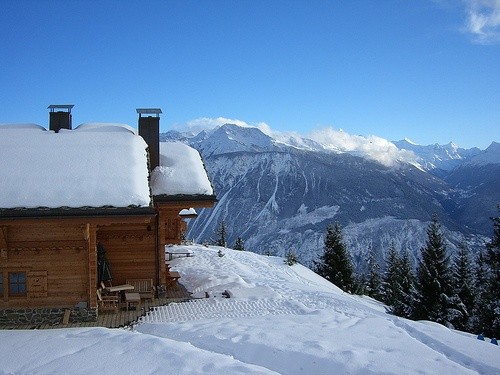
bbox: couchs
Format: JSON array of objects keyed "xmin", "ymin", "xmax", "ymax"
[{"xmin": 125, "ymin": 279, "xmax": 156, "ymax": 302}]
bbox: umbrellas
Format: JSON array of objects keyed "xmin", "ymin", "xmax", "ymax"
[{"xmin": 97, "ymin": 242, "xmax": 113, "ymax": 287}]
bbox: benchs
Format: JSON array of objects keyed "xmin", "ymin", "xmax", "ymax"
[{"xmin": 165, "ymin": 264, "xmax": 180, "ymax": 291}]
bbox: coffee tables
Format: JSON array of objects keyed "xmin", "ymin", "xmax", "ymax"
[{"xmin": 125, "ymin": 293, "xmax": 141, "ymax": 312}]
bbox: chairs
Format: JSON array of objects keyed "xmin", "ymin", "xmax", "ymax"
[{"xmin": 96, "ymin": 281, "xmax": 121, "ymax": 315}]
